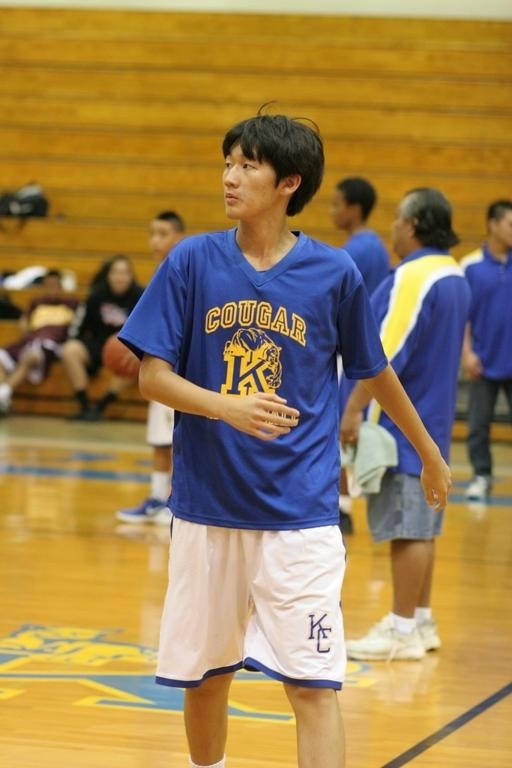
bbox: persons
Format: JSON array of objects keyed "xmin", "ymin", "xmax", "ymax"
[
  {"xmin": 344, "ymin": 187, "xmax": 466, "ymax": 661},
  {"xmin": 330, "ymin": 177, "xmax": 389, "ymax": 533},
  {"xmin": 116, "ymin": 208, "xmax": 189, "ymax": 527},
  {"xmin": 63, "ymin": 257, "xmax": 150, "ymax": 421},
  {"xmin": 460, "ymin": 194, "xmax": 511, "ymax": 499},
  {"xmin": 118, "ymin": 100, "xmax": 452, "ymax": 768},
  {"xmin": 1, "ymin": 268, "xmax": 82, "ymax": 414}
]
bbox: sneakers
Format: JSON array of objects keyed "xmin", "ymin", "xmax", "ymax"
[
  {"xmin": 0, "ymin": 383, "xmax": 13, "ymax": 412},
  {"xmin": 345, "ymin": 616, "xmax": 424, "ymax": 661},
  {"xmin": 114, "ymin": 497, "xmax": 173, "ymax": 525},
  {"xmin": 416, "ymin": 619, "xmax": 442, "ymax": 652},
  {"xmin": 464, "ymin": 474, "xmax": 492, "ymax": 500}
]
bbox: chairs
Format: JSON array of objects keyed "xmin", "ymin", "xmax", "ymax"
[{"xmin": 0, "ymin": 8, "xmax": 512, "ymax": 454}]
